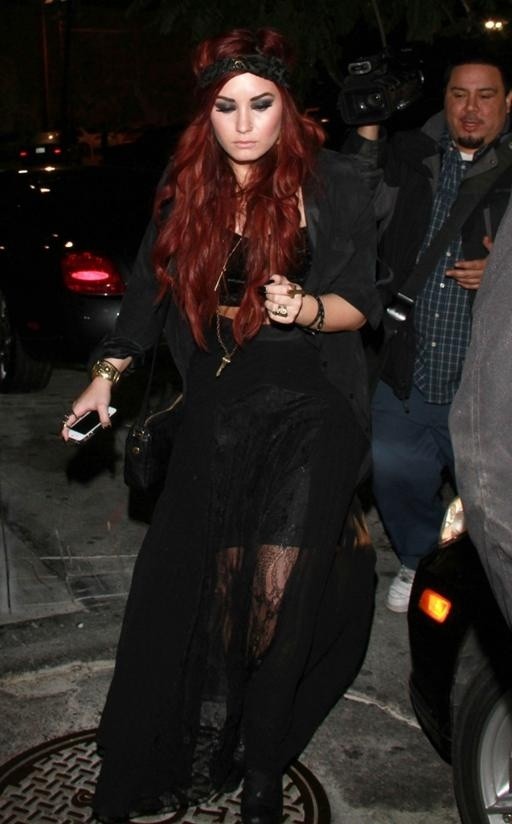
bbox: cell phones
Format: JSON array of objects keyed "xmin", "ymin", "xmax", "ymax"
[{"xmin": 67, "ymin": 405, "xmax": 118, "ymax": 445}]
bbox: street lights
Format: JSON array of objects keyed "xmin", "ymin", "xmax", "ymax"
[{"xmin": 42, "ymin": 0, "xmax": 52, "ymax": 131}]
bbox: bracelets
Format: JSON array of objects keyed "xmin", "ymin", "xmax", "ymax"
[
  {"xmin": 302, "ymin": 293, "xmax": 326, "ymax": 338},
  {"xmin": 89, "ymin": 359, "xmax": 122, "ymax": 385}
]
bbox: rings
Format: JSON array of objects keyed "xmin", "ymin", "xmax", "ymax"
[
  {"xmin": 63, "ymin": 409, "xmax": 79, "ymax": 426},
  {"xmin": 288, "ymin": 282, "xmax": 304, "ymax": 298},
  {"xmin": 273, "ymin": 304, "xmax": 288, "ymax": 317}
]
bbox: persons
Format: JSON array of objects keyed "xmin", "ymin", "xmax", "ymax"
[
  {"xmin": 343, "ymin": 35, "xmax": 510, "ymax": 611},
  {"xmin": 54, "ymin": 14, "xmax": 387, "ymax": 823}
]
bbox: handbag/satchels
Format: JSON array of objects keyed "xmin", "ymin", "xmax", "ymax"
[
  {"xmin": 122, "ymin": 422, "xmax": 161, "ymax": 498},
  {"xmin": 381, "ymin": 282, "xmax": 415, "ymax": 400}
]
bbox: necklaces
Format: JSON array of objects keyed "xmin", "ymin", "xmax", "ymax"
[
  {"xmin": 205, "ymin": 234, "xmax": 242, "ymax": 291},
  {"xmin": 216, "ymin": 284, "xmax": 241, "ymax": 378}
]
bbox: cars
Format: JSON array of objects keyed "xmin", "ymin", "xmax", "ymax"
[
  {"xmin": 1, "ymin": 168, "xmax": 157, "ymax": 394},
  {"xmin": 18, "ymin": 130, "xmax": 80, "ymax": 170}
]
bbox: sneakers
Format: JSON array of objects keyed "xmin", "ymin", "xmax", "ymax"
[{"xmin": 386, "ymin": 565, "xmax": 414, "ymax": 613}]
